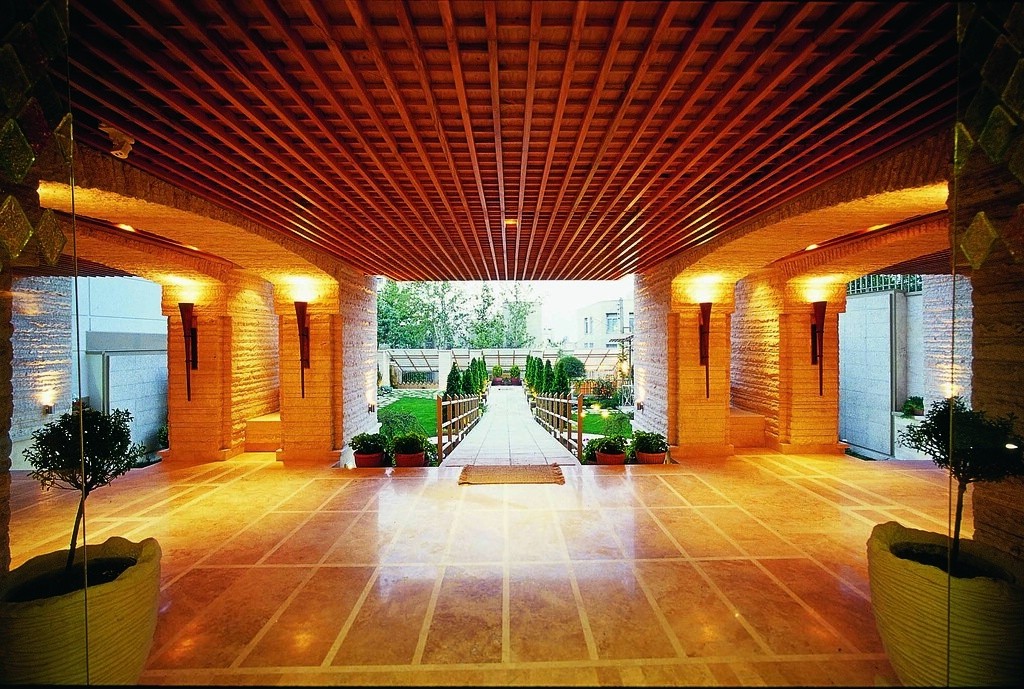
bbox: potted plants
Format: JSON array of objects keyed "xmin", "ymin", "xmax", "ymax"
[
  {"xmin": 636, "ymin": 401, "xmax": 644, "ymax": 411},
  {"xmin": 581, "ymin": 435, "xmax": 628, "ymax": 464},
  {"xmin": 1, "ymin": 397, "xmax": 161, "ymax": 688},
  {"xmin": 902, "ymin": 396, "xmax": 924, "ymax": 419},
  {"xmin": 630, "ymin": 430, "xmax": 670, "ymax": 463},
  {"xmin": 510, "ymin": 364, "xmax": 521, "ymax": 384},
  {"xmin": 393, "ymin": 432, "xmax": 435, "ymax": 466},
  {"xmin": 868, "ymin": 393, "xmax": 1021, "ymax": 687},
  {"xmin": 493, "ymin": 364, "xmax": 503, "ymax": 385},
  {"xmin": 349, "ymin": 431, "xmax": 388, "ymax": 466}
]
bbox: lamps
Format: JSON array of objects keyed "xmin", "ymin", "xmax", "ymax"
[
  {"xmin": 178, "ymin": 303, "xmax": 199, "ymax": 401},
  {"xmin": 697, "ymin": 301, "xmax": 715, "ymax": 399},
  {"xmin": 808, "ymin": 298, "xmax": 828, "ymax": 394},
  {"xmin": 45, "ymin": 402, "xmax": 56, "ymax": 414},
  {"xmin": 369, "ymin": 401, "xmax": 376, "ymax": 413},
  {"xmin": 293, "ymin": 301, "xmax": 311, "ymax": 398}
]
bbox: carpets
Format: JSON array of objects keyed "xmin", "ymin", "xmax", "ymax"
[{"xmin": 458, "ymin": 464, "xmax": 565, "ymax": 486}]
